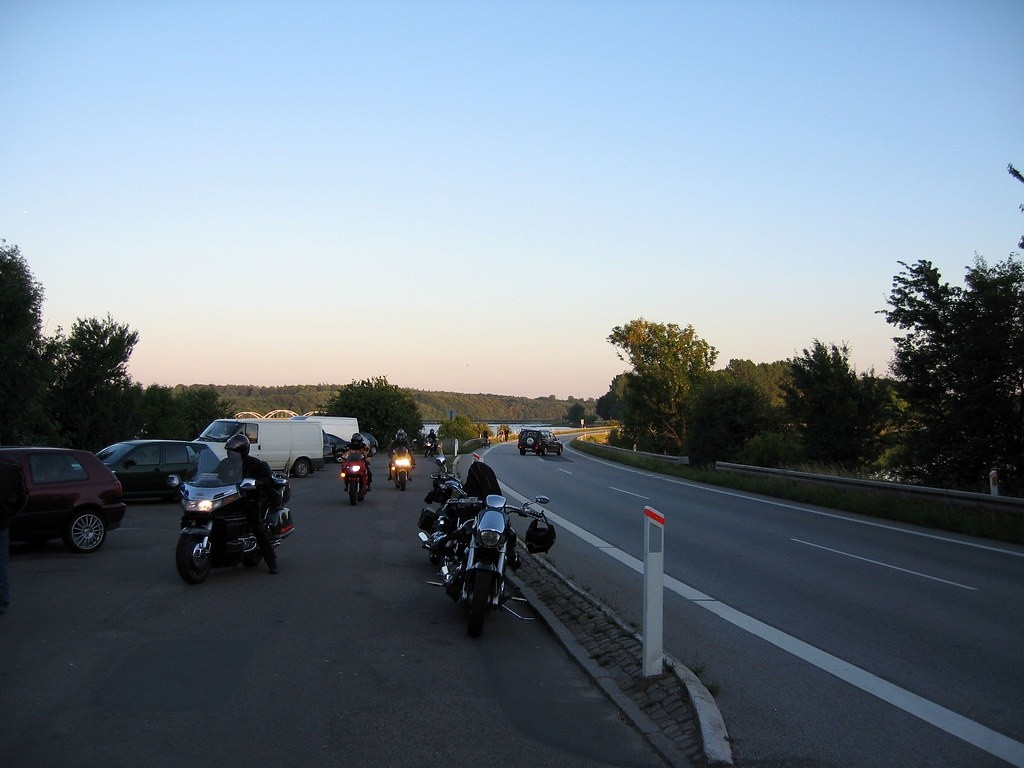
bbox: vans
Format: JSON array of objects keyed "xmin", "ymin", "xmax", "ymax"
[
  {"xmin": 200, "ymin": 418, "xmax": 325, "ymax": 478},
  {"xmin": 291, "ymin": 415, "xmax": 359, "ymax": 443}
]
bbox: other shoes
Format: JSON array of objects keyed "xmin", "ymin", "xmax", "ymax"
[
  {"xmin": 388, "ymin": 475, "xmax": 392, "ymax": 480},
  {"xmin": 367, "ymin": 485, "xmax": 371, "ymax": 492},
  {"xmin": 408, "ymin": 477, "xmax": 412, "ymax": 481},
  {"xmin": 344, "ymin": 485, "xmax": 348, "ymax": 491},
  {"xmin": 267, "ymin": 559, "xmax": 278, "ymax": 574}
]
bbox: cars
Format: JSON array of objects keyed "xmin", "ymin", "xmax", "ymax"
[
  {"xmin": 0, "ymin": 447, "xmax": 128, "ymax": 552},
  {"xmin": 323, "ymin": 433, "xmax": 352, "ymax": 463},
  {"xmin": 360, "ymin": 433, "xmax": 378, "ymax": 455}
]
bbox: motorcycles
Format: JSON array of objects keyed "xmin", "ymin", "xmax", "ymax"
[
  {"xmin": 389, "ymin": 439, "xmax": 417, "ymax": 492},
  {"xmin": 168, "ymin": 450, "xmax": 294, "ymax": 586},
  {"xmin": 417, "ymin": 455, "xmax": 550, "ymax": 637},
  {"xmin": 336, "ymin": 447, "xmax": 376, "ymax": 506}
]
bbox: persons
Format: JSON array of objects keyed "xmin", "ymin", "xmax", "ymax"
[
  {"xmin": 210, "ymin": 434, "xmax": 279, "ymax": 575},
  {"xmin": 499, "ymin": 428, "xmax": 509, "ymax": 441},
  {"xmin": 426, "ymin": 429, "xmax": 437, "ymax": 453},
  {"xmin": 482, "ymin": 430, "xmax": 488, "ymax": 444},
  {"xmin": 341, "ymin": 433, "xmax": 372, "ymax": 491},
  {"xmin": 387, "ymin": 429, "xmax": 416, "ymax": 481},
  {"xmin": 520, "ymin": 429, "xmax": 524, "ymax": 436}
]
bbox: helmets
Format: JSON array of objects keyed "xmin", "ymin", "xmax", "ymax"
[
  {"xmin": 524, "ymin": 519, "xmax": 557, "ymax": 554},
  {"xmin": 396, "ymin": 429, "xmax": 407, "ymax": 439},
  {"xmin": 224, "ymin": 434, "xmax": 250, "ymax": 459},
  {"xmin": 351, "ymin": 433, "xmax": 362, "ymax": 445},
  {"xmin": 430, "ymin": 429, "xmax": 434, "ymax": 434}
]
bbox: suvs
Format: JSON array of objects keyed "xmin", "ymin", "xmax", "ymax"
[
  {"xmin": 71, "ymin": 439, "xmax": 221, "ymax": 504},
  {"xmin": 518, "ymin": 428, "xmax": 563, "ymax": 456},
  {"xmin": 322, "ymin": 429, "xmax": 334, "ymax": 461}
]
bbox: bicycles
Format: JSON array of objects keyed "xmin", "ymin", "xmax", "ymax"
[{"xmin": 505, "ymin": 434, "xmax": 509, "ymax": 442}]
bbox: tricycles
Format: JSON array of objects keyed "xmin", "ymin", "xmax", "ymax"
[{"xmin": 481, "ymin": 439, "xmax": 491, "ymax": 449}]
